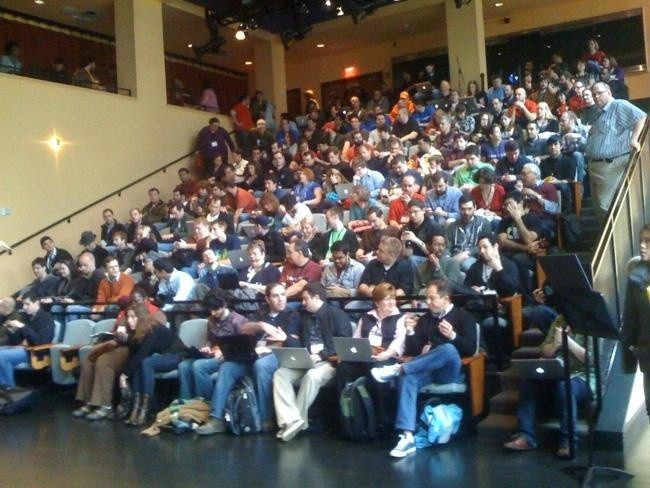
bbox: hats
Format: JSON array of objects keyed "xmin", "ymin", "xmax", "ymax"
[
  {"xmin": 79, "ymin": 230, "xmax": 97, "ymax": 245},
  {"xmin": 254, "ymin": 214, "xmax": 273, "ymax": 225},
  {"xmin": 320, "ymin": 121, "xmax": 337, "ymax": 130},
  {"xmin": 399, "ymin": 90, "xmax": 409, "ymax": 99}
]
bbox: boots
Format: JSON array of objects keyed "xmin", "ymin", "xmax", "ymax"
[{"xmin": 123, "ymin": 391, "xmax": 152, "ymax": 427}]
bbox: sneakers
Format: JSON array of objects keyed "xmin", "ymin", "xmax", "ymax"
[
  {"xmin": 276, "ymin": 427, "xmax": 285, "ymax": 439},
  {"xmin": 369, "ymin": 362, "xmax": 402, "ymax": 384},
  {"xmin": 70, "ymin": 404, "xmax": 98, "ymax": 418},
  {"xmin": 85, "ymin": 404, "xmax": 116, "ymax": 420},
  {"xmin": 282, "ymin": 417, "xmax": 305, "ymax": 442},
  {"xmin": 388, "ymin": 430, "xmax": 417, "ymax": 458},
  {"xmin": 195, "ymin": 417, "xmax": 228, "ymax": 436}
]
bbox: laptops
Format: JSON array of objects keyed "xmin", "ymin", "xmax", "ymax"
[
  {"xmin": 214, "ymin": 335, "xmax": 260, "ymax": 363},
  {"xmin": 334, "ymin": 183, "xmax": 354, "ymax": 200},
  {"xmin": 331, "ymin": 336, "xmax": 377, "ymax": 364},
  {"xmin": 242, "ymin": 225, "xmax": 257, "ymax": 238},
  {"xmin": 173, "ymin": 345, "xmax": 212, "ymax": 360},
  {"xmin": 271, "ymin": 347, "xmax": 316, "ymax": 370},
  {"xmin": 226, "ymin": 249, "xmax": 250, "ymax": 269},
  {"xmin": 510, "ymin": 359, "xmax": 584, "ymax": 381},
  {"xmin": 149, "ymin": 223, "xmax": 175, "ymax": 243},
  {"xmin": 216, "ymin": 273, "xmax": 239, "ymax": 290}
]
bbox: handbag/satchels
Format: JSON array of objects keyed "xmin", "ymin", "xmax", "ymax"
[{"xmin": 414, "ymin": 396, "xmax": 472, "ymax": 449}]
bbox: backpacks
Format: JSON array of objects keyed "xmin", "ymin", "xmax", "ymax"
[
  {"xmin": 337, "ymin": 374, "xmax": 390, "ymax": 443},
  {"xmin": 223, "ymin": 373, "xmax": 262, "ymax": 436},
  {"xmin": 0, "ymin": 384, "xmax": 43, "ymax": 416}
]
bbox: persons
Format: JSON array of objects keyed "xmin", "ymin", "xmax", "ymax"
[{"xmin": 0, "ymin": 37, "xmax": 649, "ymax": 462}]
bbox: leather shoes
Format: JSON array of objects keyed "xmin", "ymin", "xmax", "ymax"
[
  {"xmin": 555, "ymin": 431, "xmax": 578, "ymax": 459},
  {"xmin": 502, "ymin": 432, "xmax": 539, "ymax": 451}
]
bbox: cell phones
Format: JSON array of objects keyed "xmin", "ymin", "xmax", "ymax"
[
  {"xmin": 382, "ymin": 195, "xmax": 387, "ymax": 198},
  {"xmin": 401, "ymin": 226, "xmax": 409, "ymax": 233}
]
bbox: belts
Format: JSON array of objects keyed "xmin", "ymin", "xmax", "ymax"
[{"xmin": 586, "ymin": 151, "xmax": 633, "ymax": 163}]
